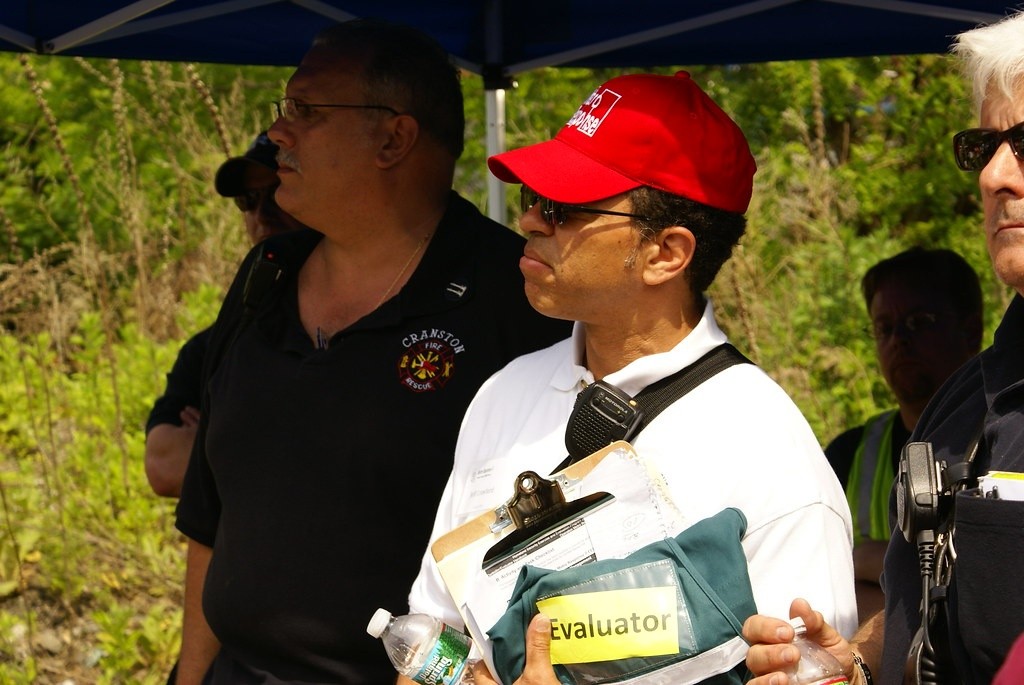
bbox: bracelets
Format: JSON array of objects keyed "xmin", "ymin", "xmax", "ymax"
[{"xmin": 849, "ymin": 652, "xmax": 872, "ymax": 685}]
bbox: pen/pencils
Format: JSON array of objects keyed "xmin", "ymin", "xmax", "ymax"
[{"xmin": 986, "ymin": 486, "xmax": 1001, "ymax": 499}]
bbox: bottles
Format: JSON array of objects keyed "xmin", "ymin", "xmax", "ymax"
[
  {"xmin": 366, "ymin": 607, "xmax": 483, "ymax": 685},
  {"xmin": 785, "ymin": 616, "xmax": 853, "ymax": 685}
]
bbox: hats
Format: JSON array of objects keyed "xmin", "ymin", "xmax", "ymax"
[
  {"xmin": 215, "ymin": 130, "xmax": 279, "ymax": 196},
  {"xmin": 488, "ymin": 69, "xmax": 757, "ymax": 214}
]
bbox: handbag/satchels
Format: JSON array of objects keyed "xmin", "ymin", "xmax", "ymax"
[{"xmin": 487, "ymin": 508, "xmax": 758, "ymax": 685}]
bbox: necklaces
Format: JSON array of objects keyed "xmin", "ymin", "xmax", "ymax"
[{"xmin": 374, "ymin": 232, "xmax": 430, "ymax": 314}]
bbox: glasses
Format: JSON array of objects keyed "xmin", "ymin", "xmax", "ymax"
[
  {"xmin": 233, "ymin": 181, "xmax": 281, "ymax": 212},
  {"xmin": 269, "ymin": 99, "xmax": 399, "ymax": 123},
  {"xmin": 863, "ymin": 310, "xmax": 964, "ymax": 339},
  {"xmin": 953, "ymin": 122, "xmax": 1024, "ymax": 171},
  {"xmin": 520, "ymin": 184, "xmax": 650, "ymax": 224}
]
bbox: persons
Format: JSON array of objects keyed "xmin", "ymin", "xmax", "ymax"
[
  {"xmin": 742, "ymin": 10, "xmax": 1024, "ymax": 685},
  {"xmin": 164, "ymin": 24, "xmax": 576, "ymax": 685},
  {"xmin": 394, "ymin": 71, "xmax": 857, "ymax": 685},
  {"xmin": 143, "ymin": 130, "xmax": 308, "ymax": 497},
  {"xmin": 821, "ymin": 245, "xmax": 987, "ymax": 629}
]
